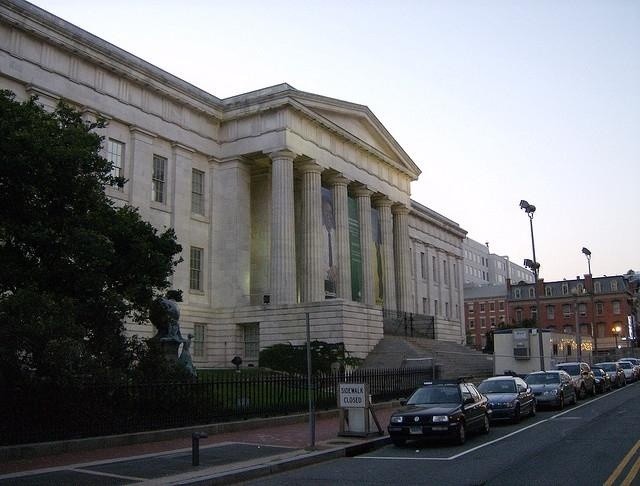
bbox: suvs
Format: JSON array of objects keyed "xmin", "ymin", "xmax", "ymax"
[{"xmin": 550, "ymin": 362, "xmax": 596, "ymax": 400}]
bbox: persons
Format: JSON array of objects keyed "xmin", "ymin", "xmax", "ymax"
[
  {"xmin": 172, "ymin": 333, "xmax": 196, "ymax": 369},
  {"xmin": 321, "ymin": 195, "xmax": 339, "ymax": 298}
]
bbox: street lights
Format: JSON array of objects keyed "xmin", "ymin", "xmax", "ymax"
[
  {"xmin": 570, "ymin": 287, "xmax": 580, "ymax": 363},
  {"xmin": 581, "ymin": 247, "xmax": 599, "ymax": 364},
  {"xmin": 519, "ymin": 199, "xmax": 545, "ymax": 372},
  {"xmin": 611, "ymin": 327, "xmax": 621, "ymax": 360}
]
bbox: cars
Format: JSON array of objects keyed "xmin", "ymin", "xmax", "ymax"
[
  {"xmin": 522, "ymin": 370, "xmax": 577, "ymax": 411},
  {"xmin": 387, "ymin": 376, "xmax": 492, "ymax": 448},
  {"xmin": 617, "ymin": 358, "xmax": 640, "ymax": 381},
  {"xmin": 477, "ymin": 376, "xmax": 536, "ymax": 424},
  {"xmin": 615, "ymin": 362, "xmax": 637, "ymax": 384},
  {"xmin": 589, "ymin": 366, "xmax": 613, "ymax": 394},
  {"xmin": 594, "ymin": 363, "xmax": 627, "ymax": 389}
]
